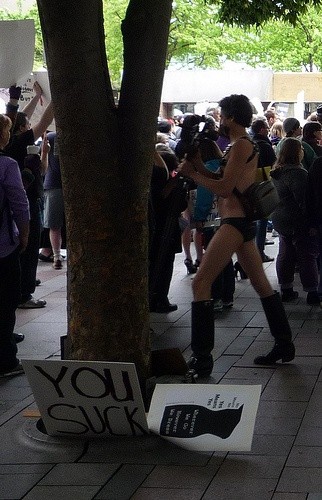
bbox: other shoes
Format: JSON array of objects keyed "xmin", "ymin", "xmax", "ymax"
[
  {"xmin": 281, "ymin": 287, "xmax": 299, "ymax": 301},
  {"xmin": 20, "ymin": 297, "xmax": 46, "ymax": 308},
  {"xmin": 306, "ymin": 291, "xmax": 320, "ymax": 306},
  {"xmin": 261, "ymin": 253, "xmax": 274, "ymax": 263},
  {"xmin": 15, "ymin": 332, "xmax": 24, "ymax": 343},
  {"xmin": 2, "ymin": 363, "xmax": 26, "ymax": 376},
  {"xmin": 212, "ymin": 297, "xmax": 234, "ymax": 311},
  {"xmin": 152, "ymin": 300, "xmax": 178, "ymax": 314},
  {"xmin": 53, "ymin": 255, "xmax": 63, "ymax": 269},
  {"xmin": 40, "ymin": 252, "xmax": 66, "ymax": 261},
  {"xmin": 35, "ymin": 278, "xmax": 41, "ymax": 285}
]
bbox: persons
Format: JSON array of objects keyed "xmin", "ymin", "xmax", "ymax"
[{"xmin": 0, "ymin": 80, "xmax": 322, "ymax": 377}]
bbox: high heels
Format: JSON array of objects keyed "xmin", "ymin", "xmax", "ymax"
[
  {"xmin": 184, "ymin": 257, "xmax": 196, "ymax": 273},
  {"xmin": 194, "ymin": 260, "xmax": 201, "ymax": 267},
  {"xmin": 236, "ymin": 262, "xmax": 247, "ymax": 281}
]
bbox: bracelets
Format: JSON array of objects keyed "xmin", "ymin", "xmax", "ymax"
[{"xmin": 193, "ymin": 163, "xmax": 204, "ymax": 168}]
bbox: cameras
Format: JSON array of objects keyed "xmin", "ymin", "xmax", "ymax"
[{"xmin": 178, "ymin": 115, "xmax": 220, "ymax": 162}]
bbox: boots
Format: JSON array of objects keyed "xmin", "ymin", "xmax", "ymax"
[
  {"xmin": 186, "ymin": 300, "xmax": 217, "ymax": 374},
  {"xmin": 252, "ymin": 291, "xmax": 296, "ymax": 366}
]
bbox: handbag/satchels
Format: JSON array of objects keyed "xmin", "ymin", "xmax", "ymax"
[{"xmin": 222, "ymin": 142, "xmax": 281, "ymax": 222}]
